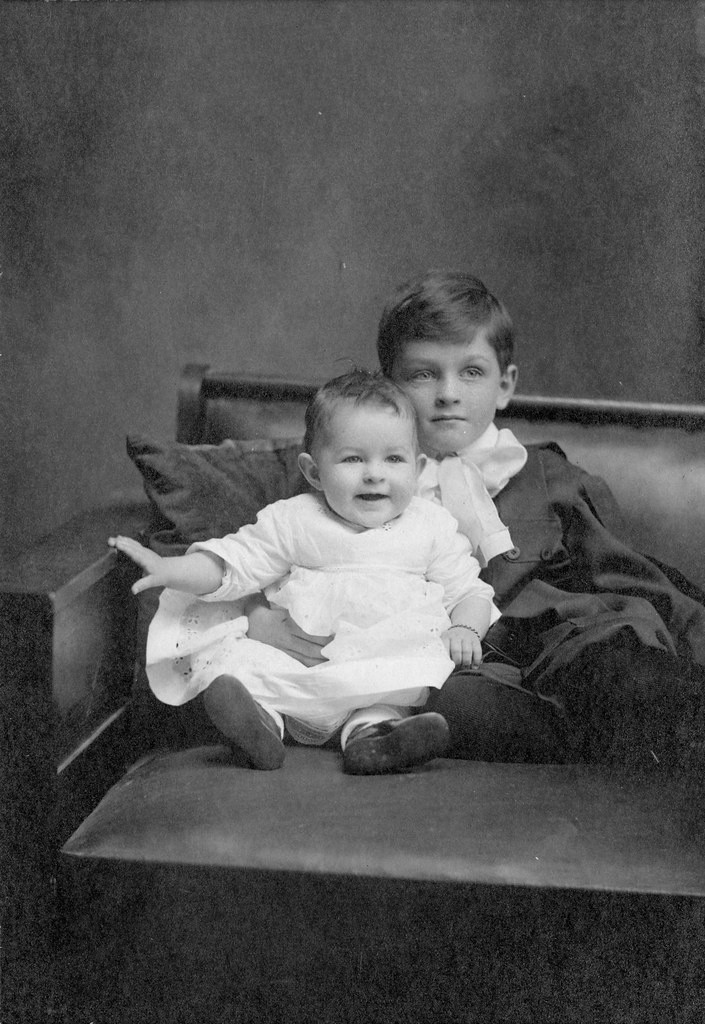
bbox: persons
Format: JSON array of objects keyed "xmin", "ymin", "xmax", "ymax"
[
  {"xmin": 106, "ymin": 370, "xmax": 495, "ymax": 775},
  {"xmin": 242, "ymin": 268, "xmax": 704, "ymax": 764}
]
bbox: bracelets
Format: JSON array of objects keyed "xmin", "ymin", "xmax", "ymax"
[{"xmin": 448, "ymin": 625, "xmax": 480, "ymax": 639}]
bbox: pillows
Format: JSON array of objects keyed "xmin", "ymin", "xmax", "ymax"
[{"xmin": 122, "ymin": 435, "xmax": 313, "ymax": 760}]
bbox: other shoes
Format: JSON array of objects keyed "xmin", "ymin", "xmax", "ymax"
[
  {"xmin": 342, "ymin": 713, "xmax": 451, "ymax": 773},
  {"xmin": 204, "ymin": 674, "xmax": 287, "ymax": 771}
]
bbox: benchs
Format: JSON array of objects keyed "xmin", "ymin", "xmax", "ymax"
[{"xmin": 0, "ymin": 356, "xmax": 705, "ymax": 1024}]
